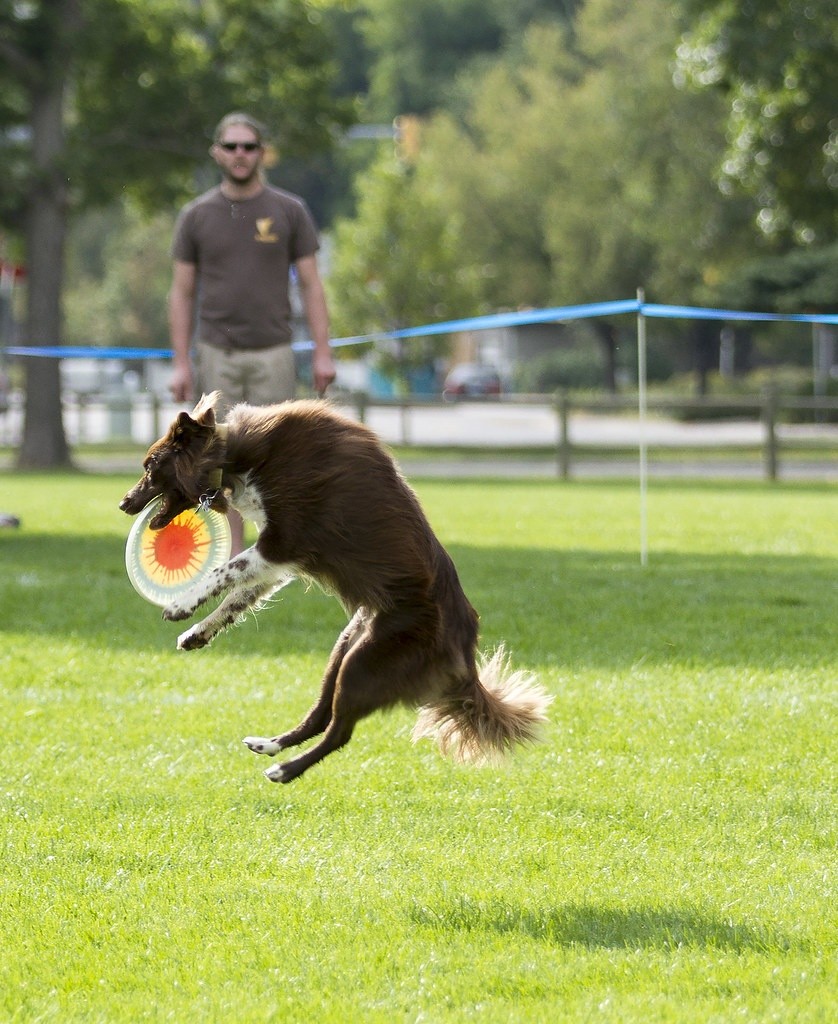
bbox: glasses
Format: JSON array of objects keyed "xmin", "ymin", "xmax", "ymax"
[{"xmin": 219, "ymin": 142, "xmax": 259, "ymax": 152}]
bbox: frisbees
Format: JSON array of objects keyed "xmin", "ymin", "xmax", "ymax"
[{"xmin": 123, "ymin": 493, "xmax": 233, "ymax": 610}]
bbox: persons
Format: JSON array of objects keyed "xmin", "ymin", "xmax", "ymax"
[{"xmin": 169, "ymin": 111, "xmax": 336, "ymax": 560}]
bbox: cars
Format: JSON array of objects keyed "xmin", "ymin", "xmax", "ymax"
[{"xmin": 441, "ymin": 361, "xmax": 501, "ymax": 405}]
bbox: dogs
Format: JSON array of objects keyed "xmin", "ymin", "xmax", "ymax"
[{"xmin": 119, "ymin": 390, "xmax": 555, "ymax": 785}]
